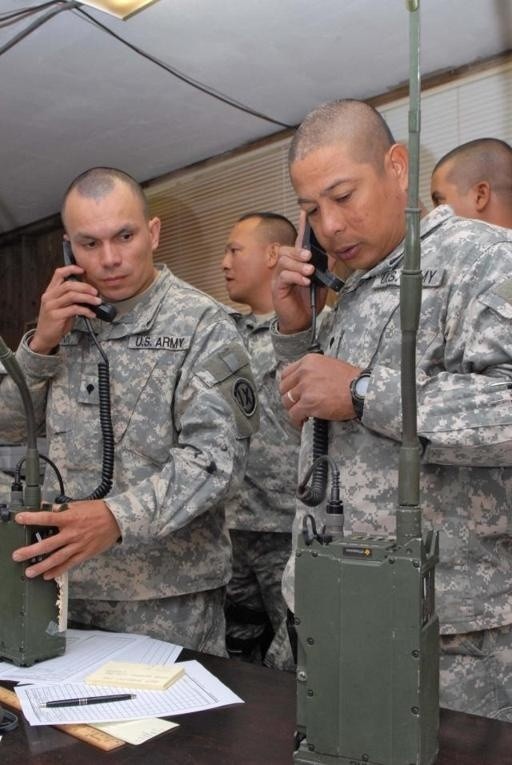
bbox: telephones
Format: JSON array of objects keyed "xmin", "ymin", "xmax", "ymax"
[
  {"xmin": 63, "ymin": 239, "xmax": 117, "ymax": 322},
  {"xmin": 301, "ymin": 222, "xmax": 346, "ymax": 292}
]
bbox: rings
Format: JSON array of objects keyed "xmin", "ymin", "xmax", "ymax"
[{"xmin": 286, "ymin": 391, "xmax": 295, "ymax": 403}]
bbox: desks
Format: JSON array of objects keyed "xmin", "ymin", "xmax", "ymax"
[{"xmin": 0, "ymin": 618, "xmax": 512, "ymax": 765}]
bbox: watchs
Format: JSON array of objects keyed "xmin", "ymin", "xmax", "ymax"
[{"xmin": 350, "ymin": 369, "xmax": 375, "ymax": 423}]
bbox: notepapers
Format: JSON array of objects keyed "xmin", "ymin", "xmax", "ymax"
[{"xmin": 86, "ymin": 659, "xmax": 187, "ymax": 690}]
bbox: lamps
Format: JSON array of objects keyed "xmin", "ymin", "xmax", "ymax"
[{"xmin": 72, "ymin": 0, "xmax": 162, "ymax": 21}]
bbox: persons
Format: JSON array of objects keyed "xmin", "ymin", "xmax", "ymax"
[
  {"xmin": 429, "ymin": 137, "xmax": 512, "ymax": 229},
  {"xmin": 221, "ymin": 213, "xmax": 336, "ymax": 666},
  {"xmin": 270, "ymin": 99, "xmax": 512, "ymax": 722},
  {"xmin": 0, "ymin": 164, "xmax": 257, "ymax": 658}
]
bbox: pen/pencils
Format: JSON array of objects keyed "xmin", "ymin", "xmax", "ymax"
[{"xmin": 40, "ymin": 693, "xmax": 137, "ymax": 708}]
